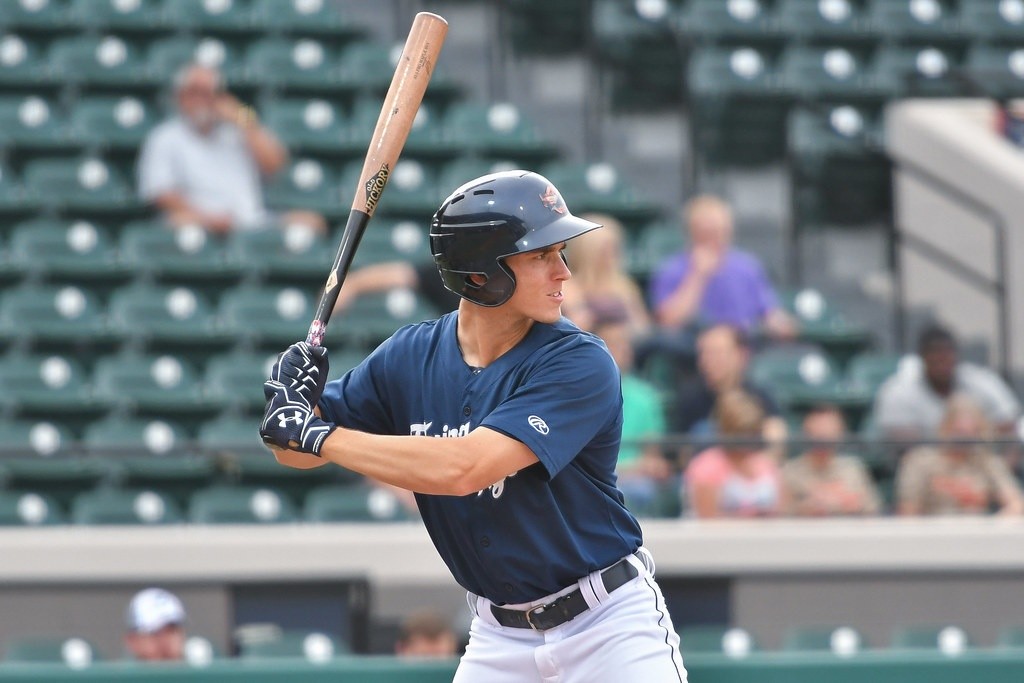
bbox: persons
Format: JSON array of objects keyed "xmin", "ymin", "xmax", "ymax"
[
  {"xmin": 258, "ymin": 172, "xmax": 689, "ymax": 683},
  {"xmin": 120, "ymin": 587, "xmax": 187, "ymax": 664},
  {"xmin": 392, "ymin": 605, "xmax": 460, "ymax": 661},
  {"xmin": 556, "ymin": 194, "xmax": 1022, "ymax": 523},
  {"xmin": 136, "ymin": 62, "xmax": 330, "ymax": 255}
]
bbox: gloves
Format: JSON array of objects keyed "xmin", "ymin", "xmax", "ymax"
[
  {"xmin": 270, "ymin": 341, "xmax": 329, "ymax": 409},
  {"xmin": 259, "ymin": 380, "xmax": 337, "ymax": 457}
]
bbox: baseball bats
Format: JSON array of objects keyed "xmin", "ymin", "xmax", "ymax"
[{"xmin": 259, "ymin": 7, "xmax": 454, "ymax": 457}]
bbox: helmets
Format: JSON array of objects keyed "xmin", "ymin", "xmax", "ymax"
[{"xmin": 429, "ymin": 170, "xmax": 603, "ymax": 307}]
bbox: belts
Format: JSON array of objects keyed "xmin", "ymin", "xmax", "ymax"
[{"xmin": 475, "ymin": 551, "xmax": 648, "ymax": 632}]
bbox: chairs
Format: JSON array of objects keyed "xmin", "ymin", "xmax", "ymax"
[{"xmin": 0, "ymin": 0, "xmax": 1024, "ymax": 528}]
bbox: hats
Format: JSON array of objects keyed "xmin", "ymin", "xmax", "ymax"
[{"xmin": 128, "ymin": 589, "xmax": 182, "ymax": 635}]
